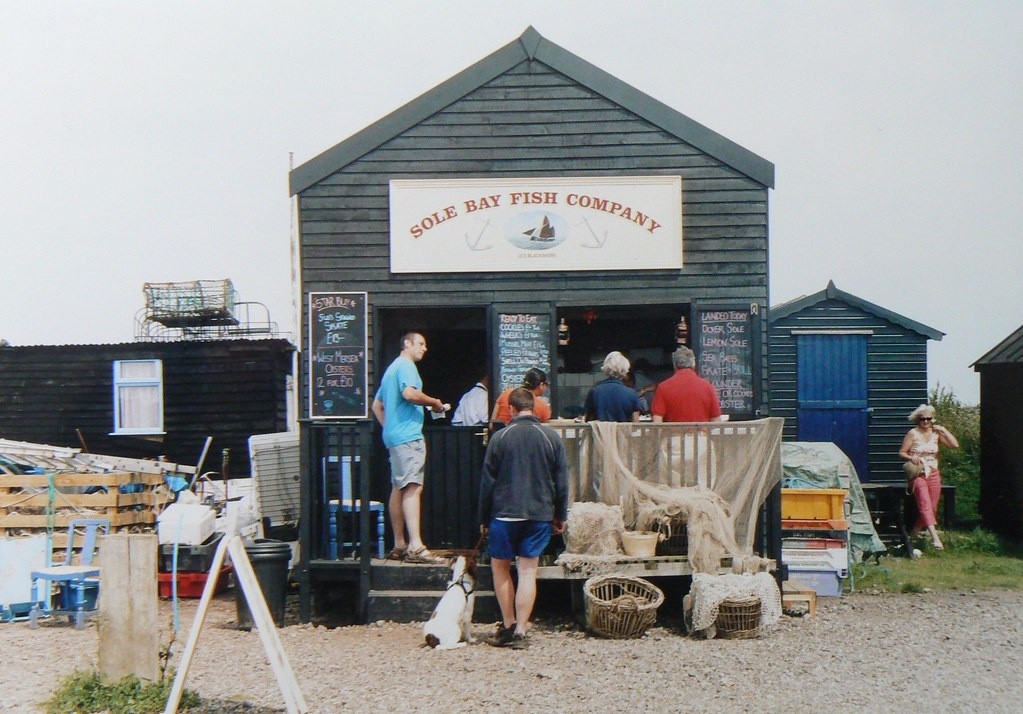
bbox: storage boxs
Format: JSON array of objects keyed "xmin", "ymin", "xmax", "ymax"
[
  {"xmin": 157, "ymin": 504, "xmax": 233, "ymax": 599},
  {"xmin": 781, "ymin": 488, "xmax": 847, "ymax": 520},
  {"xmin": 781, "ymin": 536, "xmax": 848, "ymax": 597}
]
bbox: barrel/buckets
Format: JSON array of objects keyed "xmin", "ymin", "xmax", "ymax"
[
  {"xmin": 57, "ymin": 581, "xmax": 100, "ymax": 622},
  {"xmin": 621, "ymin": 530, "xmax": 658, "ymax": 557},
  {"xmin": 229, "ymin": 542, "xmax": 291, "ymax": 629}
]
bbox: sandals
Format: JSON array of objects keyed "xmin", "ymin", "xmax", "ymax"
[
  {"xmin": 389, "ymin": 547, "xmax": 408, "ymax": 562},
  {"xmin": 404, "ymin": 544, "xmax": 447, "ymax": 565}
]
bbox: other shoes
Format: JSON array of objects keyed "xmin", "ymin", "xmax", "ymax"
[
  {"xmin": 486, "ymin": 620, "xmax": 518, "ymax": 649},
  {"xmin": 911, "ymin": 533, "xmax": 925, "ymax": 540},
  {"xmin": 510, "ymin": 631, "xmax": 533, "ymax": 650},
  {"xmin": 933, "ymin": 541, "xmax": 945, "ymax": 552}
]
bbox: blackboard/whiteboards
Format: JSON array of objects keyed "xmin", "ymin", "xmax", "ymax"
[
  {"xmin": 691, "ymin": 302, "xmax": 763, "ymax": 420},
  {"xmin": 308, "ymin": 292, "xmax": 368, "ymax": 419},
  {"xmin": 491, "ymin": 307, "xmax": 558, "ymax": 419}
]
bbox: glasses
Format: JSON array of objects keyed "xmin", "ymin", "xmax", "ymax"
[
  {"xmin": 543, "ymin": 381, "xmax": 551, "ymax": 386},
  {"xmin": 918, "ymin": 417, "xmax": 934, "ymax": 421}
]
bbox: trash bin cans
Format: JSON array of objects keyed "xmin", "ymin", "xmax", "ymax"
[{"xmin": 229, "ymin": 538, "xmax": 292, "ymax": 631}]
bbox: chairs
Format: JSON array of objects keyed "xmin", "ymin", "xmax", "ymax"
[
  {"xmin": 319, "ymin": 455, "xmax": 385, "ymax": 560},
  {"xmin": 30, "ymin": 518, "xmax": 111, "ymax": 629}
]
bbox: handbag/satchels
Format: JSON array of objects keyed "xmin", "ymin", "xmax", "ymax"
[{"xmin": 902, "ymin": 460, "xmax": 926, "ymax": 497}]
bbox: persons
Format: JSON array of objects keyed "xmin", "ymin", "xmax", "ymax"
[
  {"xmin": 450, "ymin": 366, "xmax": 490, "ymax": 426},
  {"xmin": 651, "ymin": 347, "xmax": 720, "ymax": 491},
  {"xmin": 582, "ymin": 351, "xmax": 643, "ymax": 423},
  {"xmin": 476, "ymin": 387, "xmax": 568, "ymax": 650},
  {"xmin": 490, "ymin": 368, "xmax": 551, "ymax": 422},
  {"xmin": 371, "ymin": 331, "xmax": 446, "ymax": 564},
  {"xmin": 899, "ymin": 404, "xmax": 959, "ymax": 552},
  {"xmin": 623, "ymin": 371, "xmax": 656, "ymax": 414}
]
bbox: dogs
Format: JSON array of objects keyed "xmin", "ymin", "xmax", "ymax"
[{"xmin": 423, "ymin": 553, "xmax": 478, "ymax": 651}]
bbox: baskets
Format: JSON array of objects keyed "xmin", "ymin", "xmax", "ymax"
[
  {"xmin": 585, "ymin": 573, "xmax": 664, "ymax": 643},
  {"xmin": 689, "ymin": 583, "xmax": 767, "ymax": 640}
]
